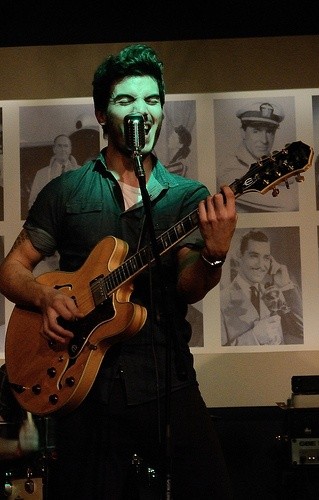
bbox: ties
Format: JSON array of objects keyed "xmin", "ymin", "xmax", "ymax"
[
  {"xmin": 249, "ymin": 287, "xmax": 260, "ymax": 315},
  {"xmin": 61, "ymin": 165, "xmax": 66, "ymax": 174}
]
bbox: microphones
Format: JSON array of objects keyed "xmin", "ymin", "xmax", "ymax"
[
  {"xmin": 123, "ymin": 113, "xmax": 147, "ymax": 184},
  {"xmin": 262, "ymin": 281, "xmax": 280, "ymax": 316}
]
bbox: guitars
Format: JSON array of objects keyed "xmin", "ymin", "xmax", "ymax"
[{"xmin": 4, "ymin": 141, "xmax": 315, "ymax": 419}]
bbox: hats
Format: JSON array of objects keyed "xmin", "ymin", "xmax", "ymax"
[{"xmin": 236, "ymin": 99, "xmax": 286, "ymax": 129}]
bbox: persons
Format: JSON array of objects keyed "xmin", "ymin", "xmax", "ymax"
[
  {"xmin": 220, "ymin": 230, "xmax": 304, "ymax": 346},
  {"xmin": 1, "ymin": 45, "xmax": 239, "ymax": 500},
  {"xmin": 218, "ymin": 102, "xmax": 298, "ymax": 211},
  {"xmin": 168, "ymin": 124, "xmax": 192, "ymax": 164},
  {"xmin": 28, "ymin": 135, "xmax": 84, "ymax": 209}
]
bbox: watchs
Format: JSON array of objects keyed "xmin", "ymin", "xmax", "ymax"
[{"xmin": 201, "ymin": 253, "xmax": 227, "ymax": 267}]
